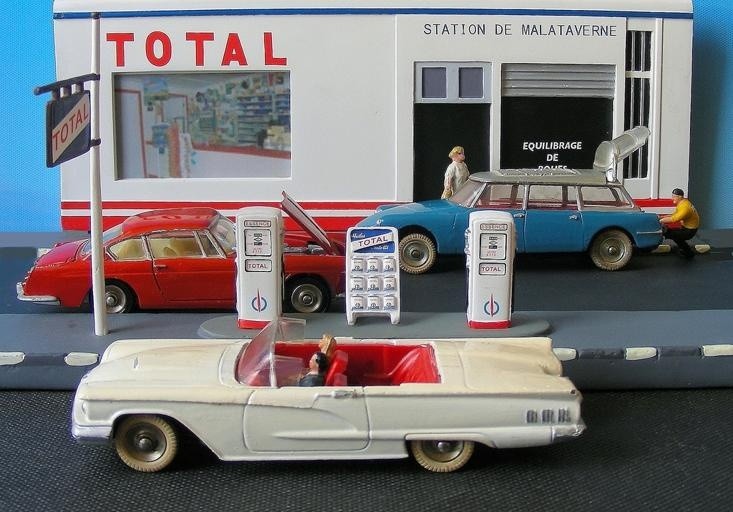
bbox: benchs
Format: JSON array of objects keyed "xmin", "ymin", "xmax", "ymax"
[{"xmin": 362, "ymin": 350, "xmax": 439, "ymax": 390}]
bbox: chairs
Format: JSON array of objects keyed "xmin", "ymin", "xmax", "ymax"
[
  {"xmin": 322, "ymin": 348, "xmax": 349, "ymax": 386},
  {"xmin": 115, "ymin": 234, "xmax": 189, "ymax": 263}
]
